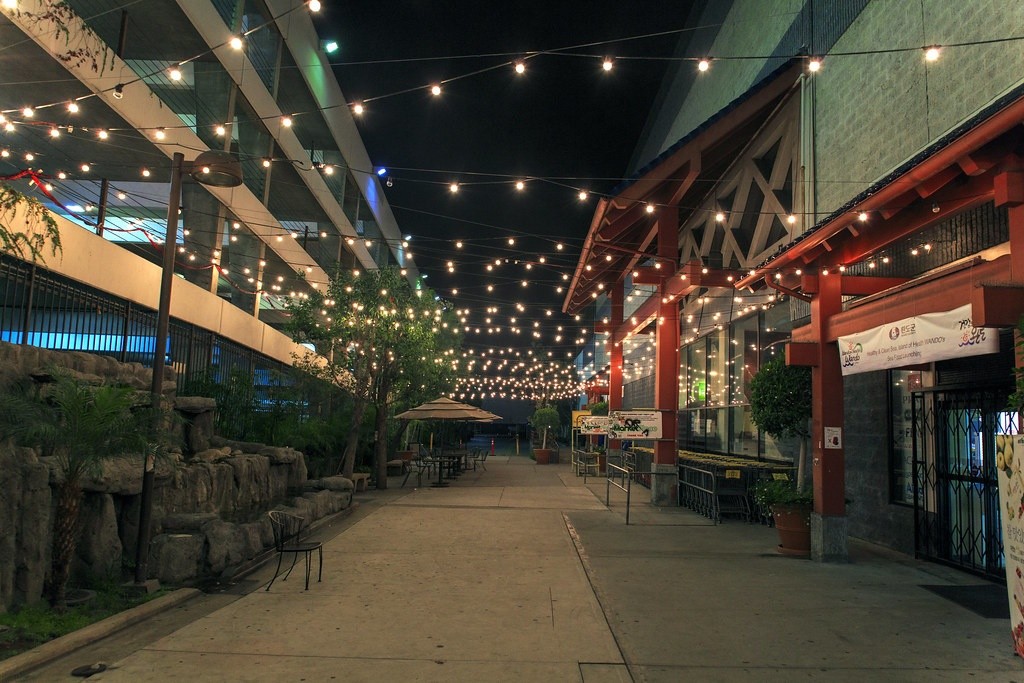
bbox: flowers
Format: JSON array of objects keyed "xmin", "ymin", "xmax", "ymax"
[
  {"xmin": 751, "ymin": 475, "xmax": 854, "ymax": 527},
  {"xmin": 591, "ymin": 443, "xmax": 607, "ymax": 455}
]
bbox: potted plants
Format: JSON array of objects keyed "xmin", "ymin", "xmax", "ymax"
[
  {"xmin": 749, "ymin": 352, "xmax": 815, "ymax": 549},
  {"xmin": 530, "ymin": 407, "xmax": 562, "ymax": 465},
  {"xmin": 589, "ymin": 400, "xmax": 610, "ymax": 472}
]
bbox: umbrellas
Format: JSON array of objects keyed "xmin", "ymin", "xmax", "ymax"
[{"xmin": 394, "ymin": 396, "xmax": 503, "ymax": 485}]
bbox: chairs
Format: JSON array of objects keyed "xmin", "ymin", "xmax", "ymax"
[
  {"xmin": 265, "ymin": 510, "xmax": 323, "ymax": 592},
  {"xmin": 400, "ymin": 446, "xmax": 489, "ymax": 489}
]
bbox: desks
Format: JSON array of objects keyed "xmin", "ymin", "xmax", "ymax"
[
  {"xmin": 423, "ymin": 459, "xmax": 453, "ymax": 488},
  {"xmin": 436, "ymin": 455, "xmax": 463, "ymax": 480},
  {"xmin": 445, "ymin": 452, "xmax": 469, "ymax": 473}
]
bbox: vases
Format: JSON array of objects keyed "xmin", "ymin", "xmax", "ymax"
[{"xmin": 395, "ymin": 451, "xmax": 414, "ymax": 466}]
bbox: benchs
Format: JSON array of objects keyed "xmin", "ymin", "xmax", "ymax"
[
  {"xmin": 337, "ymin": 472, "xmax": 370, "ymax": 494},
  {"xmin": 388, "ymin": 460, "xmax": 408, "ymax": 477}
]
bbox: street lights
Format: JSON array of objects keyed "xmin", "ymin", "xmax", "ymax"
[{"xmin": 119, "ymin": 149, "xmax": 245, "ymax": 599}]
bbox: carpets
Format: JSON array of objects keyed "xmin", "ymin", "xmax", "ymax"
[{"xmin": 917, "ymin": 583, "xmax": 1011, "ymax": 619}]
